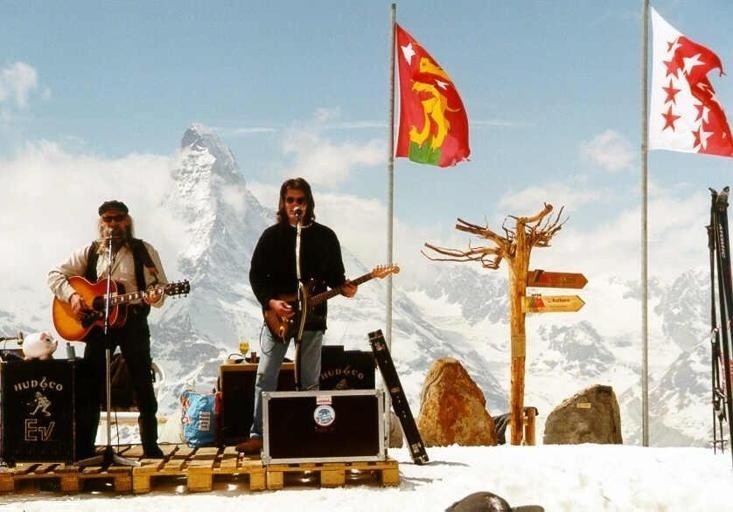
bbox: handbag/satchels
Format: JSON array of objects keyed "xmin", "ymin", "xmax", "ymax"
[{"xmin": 180, "ymin": 389, "xmax": 215, "ymax": 447}]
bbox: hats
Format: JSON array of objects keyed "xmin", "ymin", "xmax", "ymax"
[{"xmin": 99, "ymin": 200, "xmax": 128, "ymax": 215}]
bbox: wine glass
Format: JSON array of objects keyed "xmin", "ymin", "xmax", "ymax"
[{"xmin": 236, "ymin": 336, "xmax": 249, "ymax": 365}]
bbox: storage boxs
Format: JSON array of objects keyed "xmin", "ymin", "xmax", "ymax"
[{"xmin": 262, "ymin": 390, "xmax": 389, "ymax": 466}]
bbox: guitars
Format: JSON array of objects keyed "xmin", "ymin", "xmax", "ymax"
[
  {"xmin": 52, "ymin": 276, "xmax": 190, "ymax": 340},
  {"xmin": 263, "ymin": 265, "xmax": 400, "ymax": 344}
]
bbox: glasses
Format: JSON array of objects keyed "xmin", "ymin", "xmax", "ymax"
[
  {"xmin": 103, "ymin": 215, "xmax": 124, "ymax": 223},
  {"xmin": 285, "ymin": 196, "xmax": 306, "ymax": 204}
]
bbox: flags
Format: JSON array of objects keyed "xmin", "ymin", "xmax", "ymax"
[
  {"xmin": 647, "ymin": 6, "xmax": 733, "ymax": 160},
  {"xmin": 393, "ymin": 22, "xmax": 472, "ymax": 170}
]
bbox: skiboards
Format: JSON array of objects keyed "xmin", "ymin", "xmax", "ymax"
[{"xmin": 703, "ymin": 186, "xmax": 733, "ymax": 464}]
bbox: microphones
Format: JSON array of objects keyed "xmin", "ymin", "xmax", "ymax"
[
  {"xmin": 293, "ymin": 206, "xmax": 303, "ymax": 221},
  {"xmin": 106, "ymin": 227, "xmax": 115, "ymax": 236}
]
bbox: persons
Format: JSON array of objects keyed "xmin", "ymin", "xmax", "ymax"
[
  {"xmin": 44, "ymin": 200, "xmax": 171, "ymax": 460},
  {"xmin": 233, "ymin": 177, "xmax": 358, "ymax": 455}
]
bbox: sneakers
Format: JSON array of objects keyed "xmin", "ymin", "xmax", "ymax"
[
  {"xmin": 236, "ymin": 437, "xmax": 262, "ymax": 450},
  {"xmin": 143, "ymin": 444, "xmax": 165, "ymax": 458}
]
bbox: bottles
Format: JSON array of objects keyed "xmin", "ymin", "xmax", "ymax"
[
  {"xmin": 64, "ymin": 342, "xmax": 75, "ymax": 360},
  {"xmin": 250, "ymin": 352, "xmax": 257, "ymax": 363}
]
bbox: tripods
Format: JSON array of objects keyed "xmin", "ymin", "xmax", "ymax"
[{"xmin": 77, "ymin": 237, "xmax": 145, "ymax": 467}]
